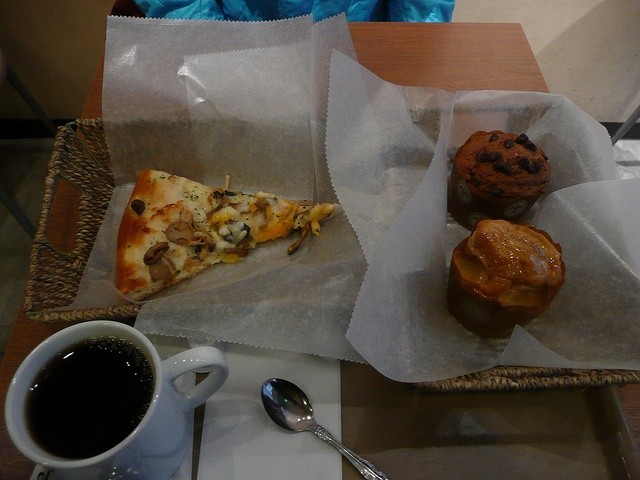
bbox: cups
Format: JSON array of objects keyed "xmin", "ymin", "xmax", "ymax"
[{"xmin": 4, "ymin": 319, "xmax": 229, "ymax": 475}]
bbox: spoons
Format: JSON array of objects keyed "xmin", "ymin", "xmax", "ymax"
[{"xmin": 261, "ymin": 376, "xmax": 389, "ymax": 476}]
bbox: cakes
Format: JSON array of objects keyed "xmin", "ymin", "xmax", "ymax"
[
  {"xmin": 445, "ymin": 217, "xmax": 566, "ymax": 338},
  {"xmin": 445, "ymin": 128, "xmax": 550, "ymax": 219}
]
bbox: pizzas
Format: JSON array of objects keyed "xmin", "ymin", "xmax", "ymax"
[{"xmin": 113, "ymin": 165, "xmax": 343, "ymax": 302}]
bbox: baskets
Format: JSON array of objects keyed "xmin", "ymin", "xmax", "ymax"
[
  {"xmin": 24, "ymin": 119, "xmax": 354, "ymax": 322},
  {"xmin": 382, "ymin": 103, "xmax": 640, "ymax": 388}
]
